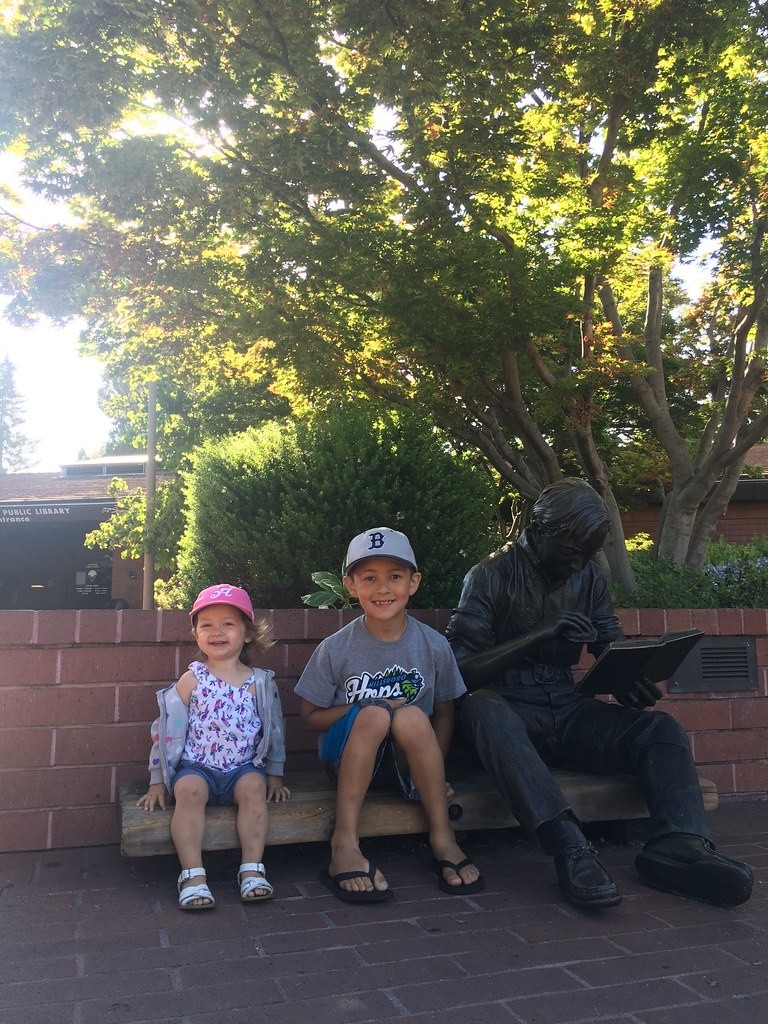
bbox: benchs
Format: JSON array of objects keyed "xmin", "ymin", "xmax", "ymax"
[{"xmin": 118, "ymin": 769, "xmax": 718, "ymax": 858}]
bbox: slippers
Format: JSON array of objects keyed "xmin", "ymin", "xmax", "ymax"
[
  {"xmin": 319, "ymin": 860, "xmax": 393, "ymax": 902},
  {"xmin": 431, "ymin": 856, "xmax": 486, "ymax": 895}
]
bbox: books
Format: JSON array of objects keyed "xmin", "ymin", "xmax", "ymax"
[{"xmin": 574, "ymin": 628, "xmax": 705, "ymax": 696}]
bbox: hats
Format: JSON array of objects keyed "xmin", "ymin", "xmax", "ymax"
[
  {"xmin": 343, "ymin": 527, "xmax": 418, "ymax": 577},
  {"xmin": 188, "ymin": 584, "xmax": 254, "ymax": 629}
]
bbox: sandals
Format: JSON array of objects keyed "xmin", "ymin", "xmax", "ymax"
[
  {"xmin": 176, "ymin": 867, "xmax": 216, "ymax": 910},
  {"xmin": 237, "ymin": 863, "xmax": 276, "ymax": 903}
]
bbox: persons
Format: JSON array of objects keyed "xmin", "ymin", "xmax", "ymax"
[
  {"xmin": 136, "ymin": 583, "xmax": 291, "ymax": 912},
  {"xmin": 294, "ymin": 528, "xmax": 484, "ymax": 905},
  {"xmin": 443, "ymin": 476, "xmax": 754, "ymax": 911}
]
